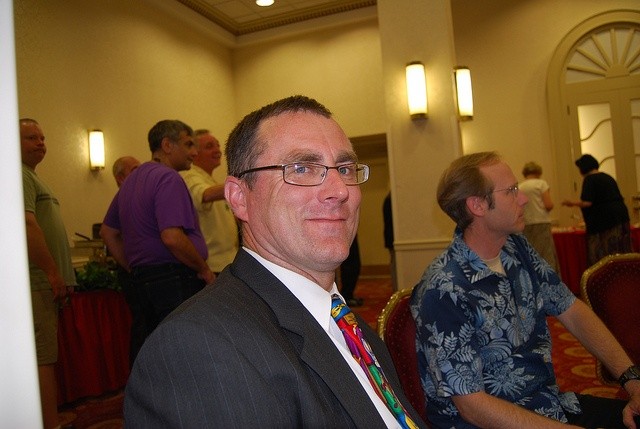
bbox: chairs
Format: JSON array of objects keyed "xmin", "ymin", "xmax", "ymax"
[
  {"xmin": 580, "ymin": 251, "xmax": 639, "ymax": 386},
  {"xmin": 378, "ymin": 286, "xmax": 428, "ymax": 425}
]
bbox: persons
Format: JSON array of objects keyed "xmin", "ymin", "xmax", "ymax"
[
  {"xmin": 561, "ymin": 154, "xmax": 632, "ymax": 268},
  {"xmin": 98, "ymin": 119, "xmax": 217, "ymax": 372},
  {"xmin": 178, "ymin": 129, "xmax": 244, "ymax": 274},
  {"xmin": 112, "ymin": 156, "xmax": 141, "ymax": 191},
  {"xmin": 338, "ymin": 236, "xmax": 362, "ymax": 308},
  {"xmin": 122, "ymin": 94, "xmax": 432, "ymax": 429},
  {"xmin": 19, "ymin": 116, "xmax": 77, "ymax": 429},
  {"xmin": 517, "ymin": 162, "xmax": 561, "ymax": 281},
  {"xmin": 408, "ymin": 151, "xmax": 639, "ymax": 429},
  {"xmin": 383, "ymin": 191, "xmax": 397, "ymax": 290}
]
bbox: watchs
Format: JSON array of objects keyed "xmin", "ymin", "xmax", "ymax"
[{"xmin": 619, "ymin": 365, "xmax": 640, "ymax": 386}]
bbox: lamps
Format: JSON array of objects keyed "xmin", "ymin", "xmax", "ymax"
[
  {"xmin": 90, "ymin": 127, "xmax": 106, "ymax": 171},
  {"xmin": 453, "ymin": 65, "xmax": 475, "ymax": 120},
  {"xmin": 404, "ymin": 60, "xmax": 429, "ymax": 121}
]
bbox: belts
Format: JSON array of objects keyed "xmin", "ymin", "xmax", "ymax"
[{"xmin": 133, "ymin": 262, "xmax": 184, "ymax": 277}]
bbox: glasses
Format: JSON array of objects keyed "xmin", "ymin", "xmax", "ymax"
[
  {"xmin": 330, "ymin": 294, "xmax": 419, "ymax": 428},
  {"xmin": 476, "ymin": 182, "xmax": 521, "ymax": 199},
  {"xmin": 237, "ymin": 162, "xmax": 370, "ymax": 187}
]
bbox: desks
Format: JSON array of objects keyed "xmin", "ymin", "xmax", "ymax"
[
  {"xmin": 51, "ymin": 289, "xmax": 129, "ymax": 407},
  {"xmin": 548, "ymin": 224, "xmax": 640, "ymax": 295}
]
